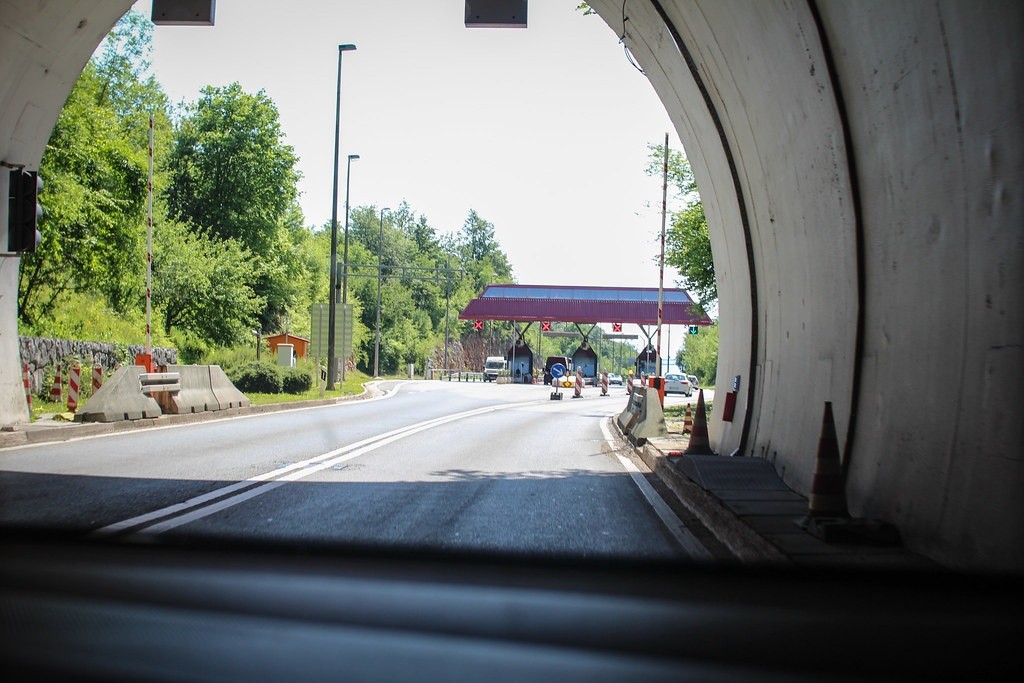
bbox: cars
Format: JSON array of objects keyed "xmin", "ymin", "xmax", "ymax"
[
  {"xmin": 664, "ymin": 373, "xmax": 693, "ymax": 397},
  {"xmin": 687, "ymin": 375, "xmax": 698, "ymax": 390},
  {"xmin": 598, "ymin": 373, "xmax": 622, "ymax": 385}
]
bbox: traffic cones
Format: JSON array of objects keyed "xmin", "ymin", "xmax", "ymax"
[
  {"xmin": 46, "ymin": 363, "xmax": 64, "ymax": 405},
  {"xmin": 683, "ymin": 389, "xmax": 718, "ymax": 456},
  {"xmin": 793, "ymin": 401, "xmax": 854, "ymax": 529},
  {"xmin": 682, "ymin": 404, "xmax": 693, "ymax": 433},
  {"xmin": 22, "ymin": 364, "xmax": 42, "ymax": 419}
]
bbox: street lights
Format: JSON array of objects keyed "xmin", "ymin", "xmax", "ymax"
[
  {"xmin": 327, "ymin": 42, "xmax": 359, "ymax": 391},
  {"xmin": 373, "ymin": 208, "xmax": 390, "ymax": 376},
  {"xmin": 344, "ymin": 154, "xmax": 359, "ymax": 302}
]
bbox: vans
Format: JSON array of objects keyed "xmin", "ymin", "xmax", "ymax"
[
  {"xmin": 483, "ymin": 356, "xmax": 505, "ymax": 382},
  {"xmin": 542, "ymin": 356, "xmax": 568, "ymax": 385}
]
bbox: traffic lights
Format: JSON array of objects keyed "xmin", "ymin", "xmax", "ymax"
[{"xmin": 7, "ymin": 170, "xmax": 46, "ymax": 255}]
